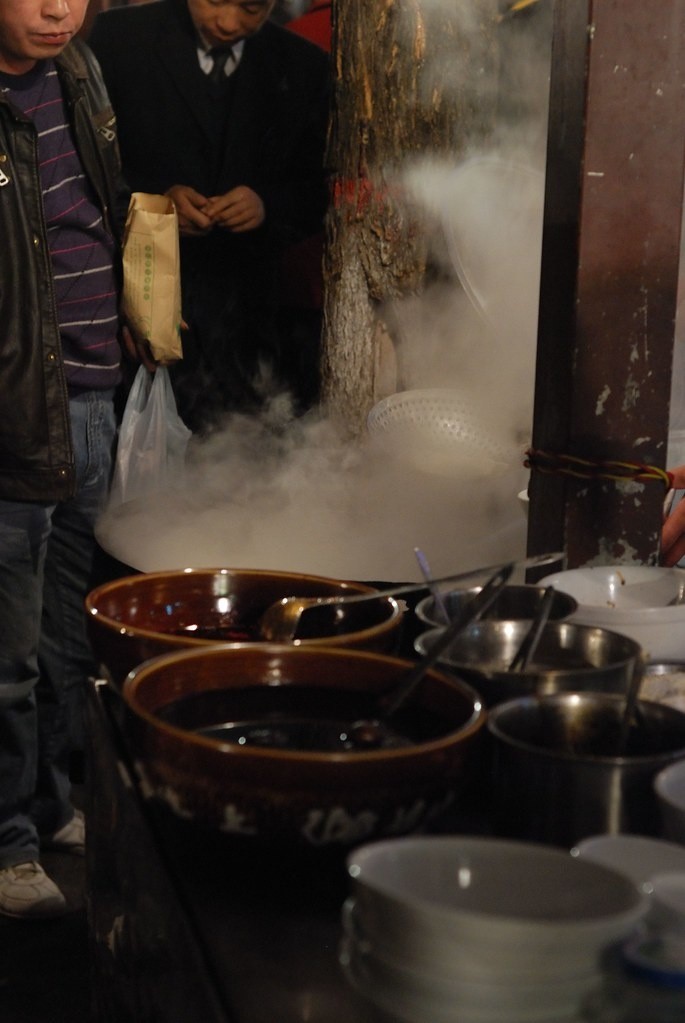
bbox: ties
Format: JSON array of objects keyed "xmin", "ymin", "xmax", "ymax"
[{"xmin": 206, "ymin": 46, "xmax": 232, "ymax": 89}]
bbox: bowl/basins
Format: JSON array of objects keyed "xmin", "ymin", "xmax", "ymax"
[
  {"xmin": 124, "ymin": 644, "xmax": 486, "ymax": 875},
  {"xmin": 538, "ymin": 565, "xmax": 685, "ymax": 663},
  {"xmin": 572, "ymin": 835, "xmax": 685, "ymax": 978},
  {"xmin": 415, "ymin": 585, "xmax": 578, "ymax": 628},
  {"xmin": 340, "ymin": 835, "xmax": 646, "ymax": 1023},
  {"xmin": 86, "ymin": 568, "xmax": 403, "ymax": 707},
  {"xmin": 414, "ymin": 619, "xmax": 643, "ymax": 712},
  {"xmin": 654, "ymin": 759, "xmax": 685, "ymax": 843},
  {"xmin": 489, "ymin": 693, "xmax": 685, "ymax": 847}
]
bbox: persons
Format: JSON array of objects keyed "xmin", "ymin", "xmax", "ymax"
[
  {"xmin": 0, "ymin": 0, "xmax": 190, "ymax": 919},
  {"xmin": 81, "ymin": 0, "xmax": 337, "ymax": 437}
]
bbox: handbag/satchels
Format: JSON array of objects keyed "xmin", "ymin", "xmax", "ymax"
[{"xmin": 108, "ymin": 361, "xmax": 192, "ymax": 511}]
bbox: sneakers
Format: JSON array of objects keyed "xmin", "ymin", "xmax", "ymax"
[
  {"xmin": 37, "ymin": 807, "xmax": 85, "ymax": 858},
  {"xmin": 0, "ymin": 860, "xmax": 67, "ymax": 921}
]
